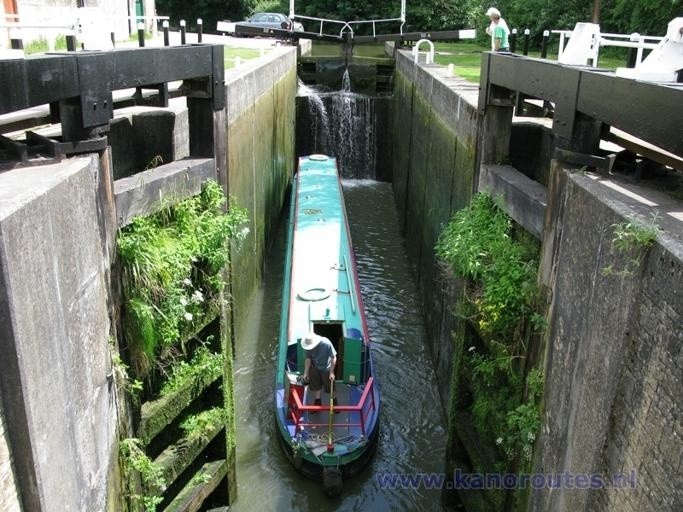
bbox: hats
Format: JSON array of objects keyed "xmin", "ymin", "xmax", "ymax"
[{"xmin": 301, "ymin": 332, "xmax": 321, "ymax": 351}]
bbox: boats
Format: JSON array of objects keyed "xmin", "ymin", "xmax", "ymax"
[{"xmin": 278, "ymin": 152, "xmax": 381, "ymax": 498}]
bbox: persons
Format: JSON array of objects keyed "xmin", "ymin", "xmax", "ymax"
[
  {"xmin": 485, "ymin": 7, "xmax": 510, "ymax": 53},
  {"xmin": 301, "ymin": 333, "xmax": 342, "ymax": 414}
]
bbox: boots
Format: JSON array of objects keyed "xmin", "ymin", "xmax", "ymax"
[
  {"xmin": 309, "ymin": 399, "xmax": 323, "ymax": 414},
  {"xmin": 333, "ymin": 397, "xmax": 340, "ymax": 414}
]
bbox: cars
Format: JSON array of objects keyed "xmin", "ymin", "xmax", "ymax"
[{"xmin": 236, "ymin": 14, "xmax": 294, "ymax": 37}]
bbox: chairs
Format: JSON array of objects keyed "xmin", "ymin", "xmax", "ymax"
[
  {"xmin": 616, "ymin": 17, "xmax": 683, "ymax": 83},
  {"xmin": 558, "ymin": 21, "xmax": 601, "ymax": 68}
]
